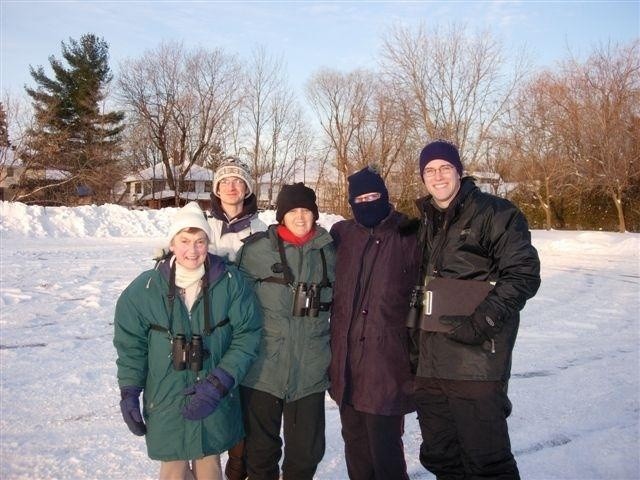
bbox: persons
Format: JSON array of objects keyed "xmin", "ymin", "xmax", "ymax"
[
  {"xmin": 233, "ymin": 183, "xmax": 338, "ymax": 479},
  {"xmin": 406, "ymin": 142, "xmax": 542, "ymax": 480},
  {"xmin": 113, "ymin": 199, "xmax": 261, "ymax": 480},
  {"xmin": 328, "ymin": 166, "xmax": 418, "ymax": 479},
  {"xmin": 203, "ymin": 156, "xmax": 268, "ymax": 262}
]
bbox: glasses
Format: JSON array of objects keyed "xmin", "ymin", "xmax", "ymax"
[
  {"xmin": 422, "ymin": 163, "xmax": 456, "ymax": 178},
  {"xmin": 219, "ymin": 179, "xmax": 244, "ymax": 185}
]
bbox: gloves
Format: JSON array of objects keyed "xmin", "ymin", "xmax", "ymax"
[
  {"xmin": 440, "ymin": 311, "xmax": 491, "ymax": 346},
  {"xmin": 181, "ymin": 368, "xmax": 233, "ymax": 421},
  {"xmin": 120, "ymin": 387, "xmax": 147, "ymax": 436}
]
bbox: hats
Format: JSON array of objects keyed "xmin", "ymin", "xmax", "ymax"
[
  {"xmin": 420, "ymin": 141, "xmax": 463, "ymax": 183},
  {"xmin": 168, "ymin": 201, "xmax": 212, "ymax": 244},
  {"xmin": 277, "ymin": 182, "xmax": 320, "ymax": 221},
  {"xmin": 348, "ymin": 167, "xmax": 389, "ymax": 227},
  {"xmin": 212, "ymin": 156, "xmax": 254, "ymax": 200}
]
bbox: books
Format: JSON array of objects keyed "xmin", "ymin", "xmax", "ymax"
[{"xmin": 420, "ymin": 275, "xmax": 499, "ymax": 333}]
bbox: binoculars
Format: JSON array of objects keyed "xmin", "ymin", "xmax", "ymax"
[
  {"xmin": 293, "ymin": 282, "xmax": 320, "ymax": 318},
  {"xmin": 172, "ymin": 333, "xmax": 203, "ymax": 371},
  {"xmin": 405, "ymin": 286, "xmax": 424, "ymax": 328}
]
